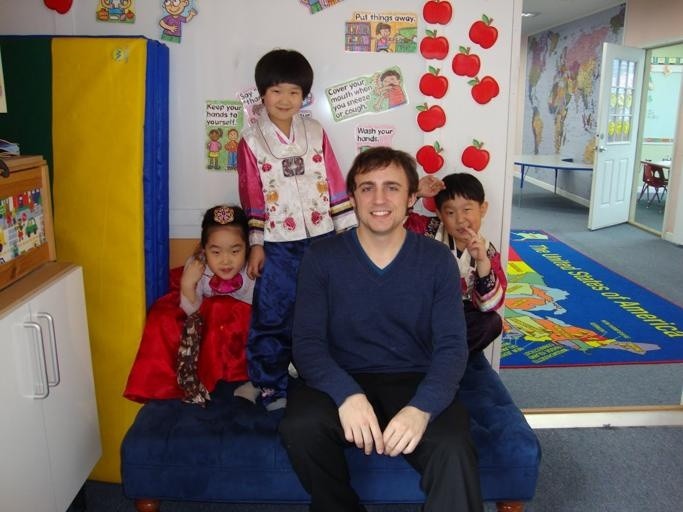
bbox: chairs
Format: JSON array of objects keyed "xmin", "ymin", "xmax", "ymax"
[{"xmin": 637, "ymin": 163, "xmax": 668, "ymax": 208}]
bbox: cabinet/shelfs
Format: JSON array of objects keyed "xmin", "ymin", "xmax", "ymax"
[
  {"xmin": 0, "ymin": 36, "xmax": 170, "ymax": 484},
  {"xmin": 0, "ymin": 264, "xmax": 103, "ymax": 512}
]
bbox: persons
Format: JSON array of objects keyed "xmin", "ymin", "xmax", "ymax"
[
  {"xmin": 236, "ymin": 48, "xmax": 358, "ymax": 412},
  {"xmin": 401, "ymin": 172, "xmax": 508, "ymax": 354},
  {"xmin": 276, "ymin": 145, "xmax": 485, "ymax": 511},
  {"xmin": 122, "ymin": 204, "xmax": 255, "ymax": 408}
]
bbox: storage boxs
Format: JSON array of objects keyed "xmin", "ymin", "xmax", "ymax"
[{"xmin": 0, "ymin": 154, "xmax": 57, "ymax": 291}]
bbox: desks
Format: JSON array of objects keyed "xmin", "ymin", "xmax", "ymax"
[
  {"xmin": 640, "ymin": 159, "xmax": 672, "ymax": 170},
  {"xmin": 514, "ymin": 155, "xmax": 594, "ymax": 195}
]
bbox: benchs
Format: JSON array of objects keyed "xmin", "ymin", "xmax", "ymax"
[{"xmin": 119, "ymin": 349, "xmax": 542, "ymax": 512}]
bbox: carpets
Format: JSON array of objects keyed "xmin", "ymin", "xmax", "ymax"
[{"xmin": 499, "ymin": 228, "xmax": 683, "ymax": 368}]
